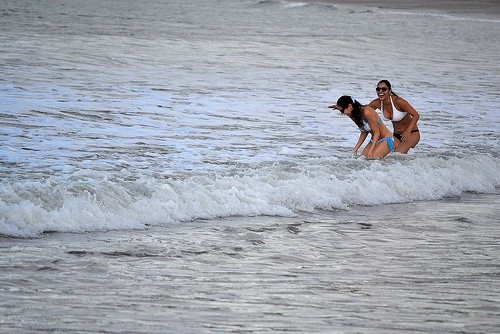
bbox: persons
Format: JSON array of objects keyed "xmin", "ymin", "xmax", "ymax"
[
  {"xmin": 327, "ymin": 79, "xmax": 421, "ymax": 154},
  {"xmin": 336, "ymin": 95, "xmax": 395, "ymax": 160}
]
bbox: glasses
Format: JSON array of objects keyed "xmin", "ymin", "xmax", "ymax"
[
  {"xmin": 340, "ymin": 108, "xmax": 346, "ymax": 113},
  {"xmin": 376, "ymin": 87, "xmax": 389, "ymax": 91}
]
